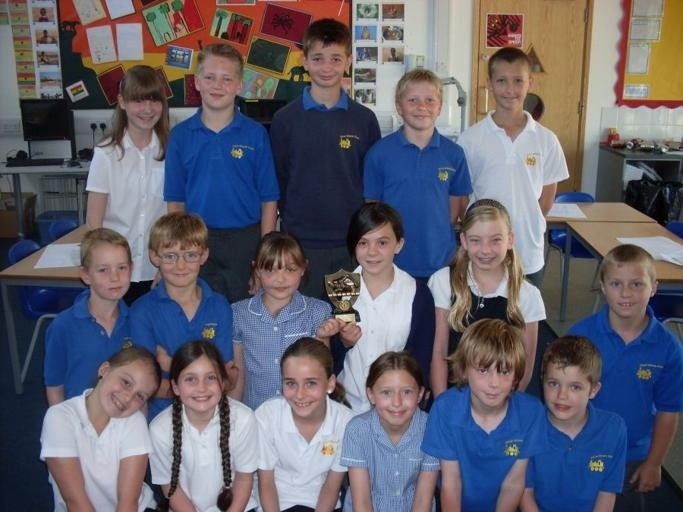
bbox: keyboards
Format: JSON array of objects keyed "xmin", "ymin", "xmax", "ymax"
[{"xmin": 5, "ymin": 157, "xmax": 65, "ymax": 166}]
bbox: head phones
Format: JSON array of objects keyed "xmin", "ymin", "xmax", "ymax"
[{"xmin": 5, "ymin": 147, "xmax": 29, "ymax": 162}]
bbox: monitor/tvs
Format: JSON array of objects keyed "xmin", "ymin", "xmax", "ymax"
[{"xmin": 18, "ymin": 98, "xmax": 72, "ymax": 142}]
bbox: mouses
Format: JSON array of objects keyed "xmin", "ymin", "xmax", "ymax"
[{"xmin": 67, "ymin": 158, "xmax": 82, "ymax": 167}]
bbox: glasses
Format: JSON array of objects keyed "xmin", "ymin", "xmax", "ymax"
[{"xmin": 152, "ymin": 247, "xmax": 204, "ymax": 264}]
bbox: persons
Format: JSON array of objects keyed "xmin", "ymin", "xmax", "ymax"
[
  {"xmin": 388, "ymin": 47, "xmax": 399, "ymax": 62},
  {"xmin": 567, "ymin": 243, "xmax": 683, "ymax": 512},
  {"xmin": 38, "ymin": 198, "xmax": 627, "ymax": 511},
  {"xmin": 39, "ymin": 51, "xmax": 47, "ymax": 65},
  {"xmin": 355, "ymin": 89, "xmax": 374, "ymax": 104},
  {"xmin": 38, "ymin": 7, "xmax": 49, "ymax": 22},
  {"xmin": 84, "ymin": 65, "xmax": 172, "ymax": 307},
  {"xmin": 361, "ymin": 26, "xmax": 370, "ymax": 40},
  {"xmin": 151, "ymin": 42, "xmax": 282, "ymax": 305},
  {"xmin": 455, "ymin": 46, "xmax": 571, "ymax": 293},
  {"xmin": 270, "ymin": 17, "xmax": 382, "ymax": 304},
  {"xmin": 361, "ymin": 67, "xmax": 473, "ymax": 286},
  {"xmin": 39, "ymin": 29, "xmax": 49, "ymax": 44}
]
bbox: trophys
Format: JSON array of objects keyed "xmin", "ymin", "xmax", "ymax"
[{"xmin": 324, "ymin": 269, "xmax": 360, "ymax": 323}]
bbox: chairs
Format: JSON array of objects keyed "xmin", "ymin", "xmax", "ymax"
[
  {"xmin": 649, "ymin": 220, "xmax": 682, "ymax": 323},
  {"xmin": 50, "ymin": 217, "xmax": 79, "ymax": 239},
  {"xmin": 548, "ymin": 193, "xmax": 603, "ymax": 288},
  {"xmin": 8, "ymin": 240, "xmax": 83, "ymax": 384}
]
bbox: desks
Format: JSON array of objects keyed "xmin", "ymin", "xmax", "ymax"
[
  {"xmin": 595, "ymin": 141, "xmax": 683, "ymax": 201},
  {"xmin": 545, "ymin": 202, "xmax": 659, "ymax": 322},
  {"xmin": 1, "ymin": 161, "xmax": 91, "ymax": 240},
  {"xmin": 0, "ymin": 225, "xmax": 91, "ymax": 395},
  {"xmin": 560, "ymin": 222, "xmax": 683, "ymax": 326}
]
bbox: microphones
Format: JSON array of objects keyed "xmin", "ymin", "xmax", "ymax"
[
  {"xmin": 88, "ymin": 122, "xmax": 98, "ymax": 153},
  {"xmin": 99, "ymin": 121, "xmax": 110, "ymax": 139}
]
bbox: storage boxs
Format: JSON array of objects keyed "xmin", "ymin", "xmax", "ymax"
[{"xmin": 1, "ymin": 191, "xmax": 37, "ymax": 238}]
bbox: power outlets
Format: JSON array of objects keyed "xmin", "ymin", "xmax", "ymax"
[
  {"xmin": 73, "ymin": 117, "xmax": 114, "ymax": 135},
  {"xmin": 1, "ymin": 116, "xmax": 22, "ymax": 138}
]
